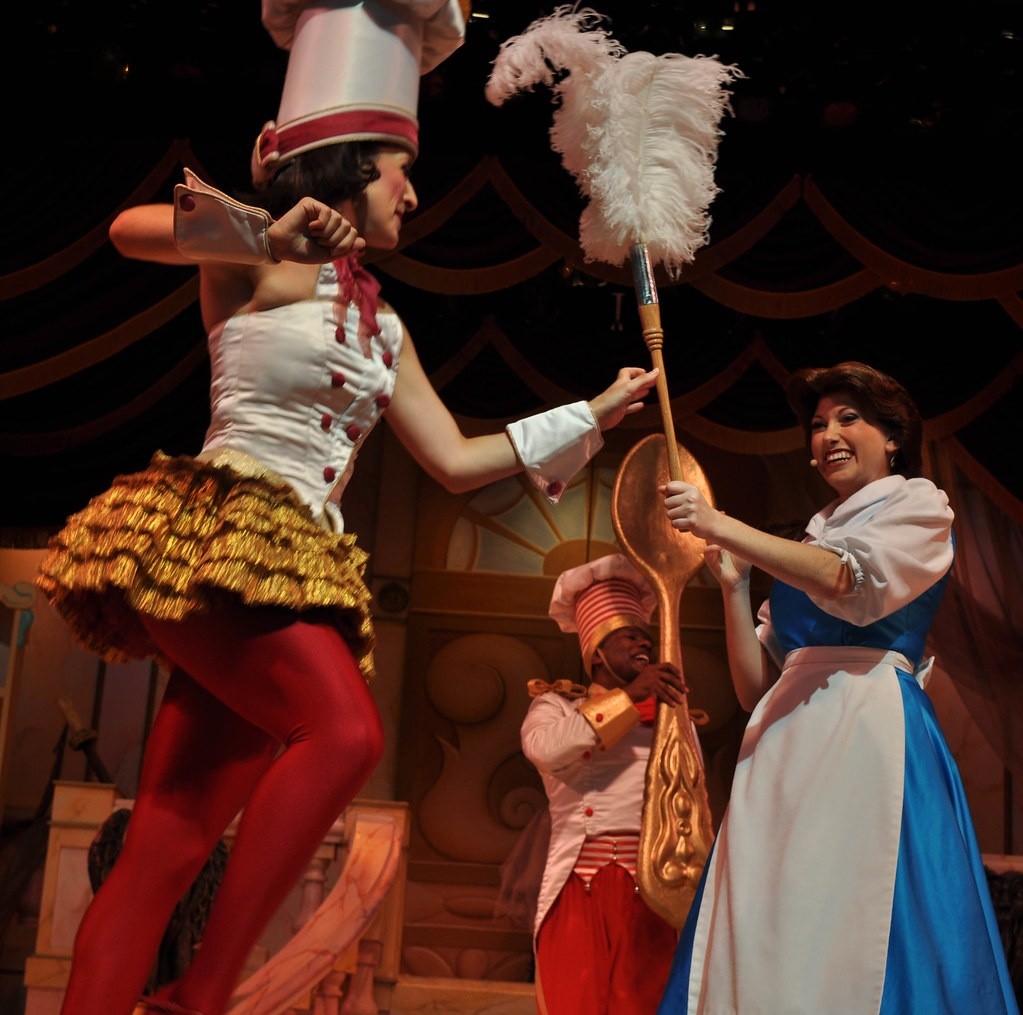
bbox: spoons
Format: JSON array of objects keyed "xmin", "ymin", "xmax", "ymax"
[{"xmin": 612, "ymin": 434, "xmax": 715, "ymax": 929}]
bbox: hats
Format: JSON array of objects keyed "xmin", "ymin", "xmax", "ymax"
[
  {"xmin": 548, "ymin": 553, "xmax": 658, "ymax": 680},
  {"xmin": 252, "ymin": 0, "xmax": 470, "ymax": 193}
]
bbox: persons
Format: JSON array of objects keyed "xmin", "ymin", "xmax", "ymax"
[
  {"xmin": 518, "ymin": 554, "xmax": 709, "ymax": 1015},
  {"xmin": 659, "ymin": 361, "xmax": 1022, "ymax": 1015},
  {"xmin": 25, "ymin": 0, "xmax": 635, "ymax": 1015}
]
